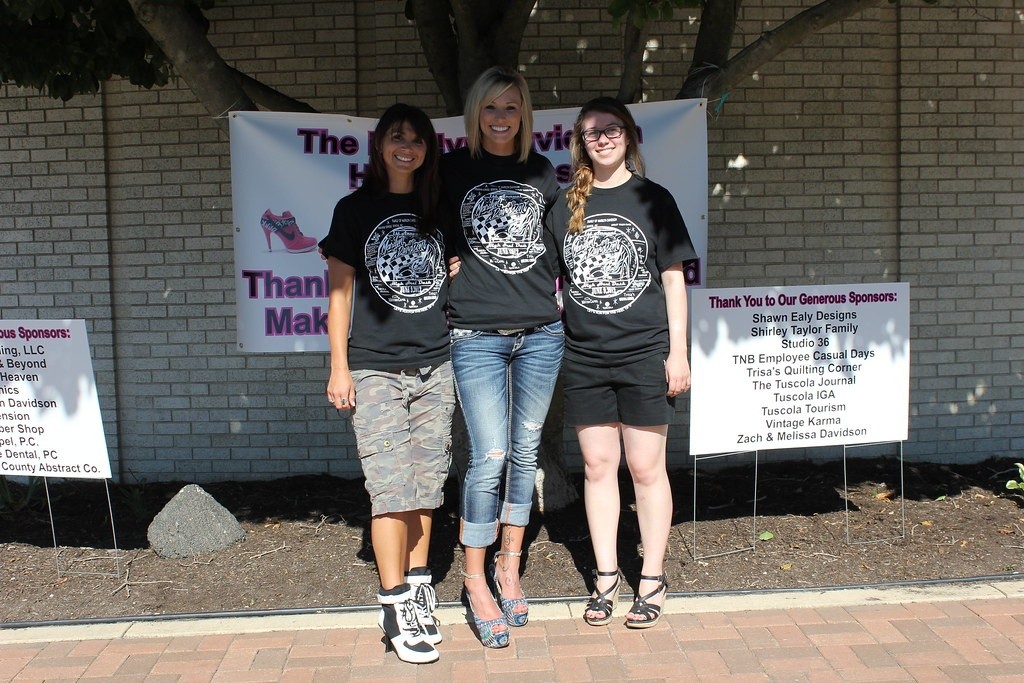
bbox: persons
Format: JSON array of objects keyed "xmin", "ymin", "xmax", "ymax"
[
  {"xmin": 317, "ymin": 67, "xmax": 564, "ymax": 647},
  {"xmin": 450, "ymin": 97, "xmax": 699, "ymax": 627},
  {"xmin": 320, "ymin": 103, "xmax": 456, "ymax": 663}
]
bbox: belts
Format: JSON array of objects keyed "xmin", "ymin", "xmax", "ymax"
[
  {"xmin": 389, "ymin": 361, "xmax": 444, "ymax": 376},
  {"xmin": 483, "ymin": 318, "xmax": 560, "ymax": 337}
]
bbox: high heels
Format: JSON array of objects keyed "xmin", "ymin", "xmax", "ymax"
[
  {"xmin": 626, "ymin": 571, "xmax": 668, "ymax": 628},
  {"xmin": 374, "ymin": 583, "xmax": 439, "ymax": 664},
  {"xmin": 492, "ymin": 550, "xmax": 529, "ymax": 627},
  {"xmin": 585, "ymin": 569, "xmax": 622, "ymax": 626},
  {"xmin": 404, "ymin": 566, "xmax": 443, "ymax": 645},
  {"xmin": 460, "ymin": 571, "xmax": 510, "ymax": 648}
]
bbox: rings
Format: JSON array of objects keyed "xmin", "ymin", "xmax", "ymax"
[
  {"xmin": 341, "ymin": 399, "xmax": 347, "ymax": 405},
  {"xmin": 329, "ymin": 400, "xmax": 334, "ymax": 403}
]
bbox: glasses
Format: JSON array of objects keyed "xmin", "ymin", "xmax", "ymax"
[{"xmin": 582, "ymin": 126, "xmax": 626, "ymax": 142}]
